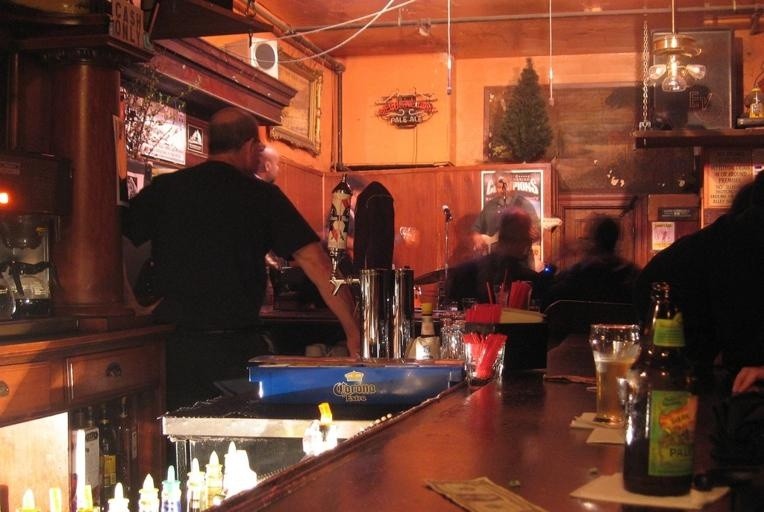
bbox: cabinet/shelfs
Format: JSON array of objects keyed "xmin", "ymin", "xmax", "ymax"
[
  {"xmin": 275, "ymin": 155, "xmax": 331, "ymax": 269},
  {"xmin": 332, "ymin": 169, "xmax": 386, "ymax": 310},
  {"xmin": 638, "ymin": 193, "xmax": 701, "ymax": 271},
  {"xmin": 1, "ymin": 319, "xmax": 167, "ymax": 490},
  {"xmin": 475, "ymin": 162, "xmax": 559, "ymax": 268},
  {"xmin": 700, "ymin": 164, "xmax": 764, "ymax": 229},
  {"xmin": 434, "ymin": 166, "xmax": 476, "ymax": 271},
  {"xmin": 552, "ymin": 190, "xmax": 638, "ymax": 273},
  {"xmin": 387, "ymin": 168, "xmax": 436, "ymax": 317}
]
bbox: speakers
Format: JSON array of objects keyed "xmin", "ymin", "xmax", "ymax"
[{"xmin": 224, "ymin": 37, "xmax": 279, "ymax": 80}]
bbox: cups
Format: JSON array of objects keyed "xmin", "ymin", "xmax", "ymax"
[
  {"xmin": 588, "ymin": 324, "xmax": 641, "ymax": 429},
  {"xmin": 464, "ymin": 342, "xmax": 505, "ymax": 386}
]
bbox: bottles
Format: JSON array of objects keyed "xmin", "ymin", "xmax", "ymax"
[
  {"xmin": 623, "ymin": 280, "xmax": 698, "ymax": 494},
  {"xmin": 749, "ymin": 87, "xmax": 764, "ymax": 118},
  {"xmin": 406, "ymin": 302, "xmax": 440, "ymax": 360},
  {"xmin": 86, "ymin": 393, "xmax": 138, "ymax": 512},
  {"xmin": 107, "ymin": 450, "xmax": 224, "ymax": 512}
]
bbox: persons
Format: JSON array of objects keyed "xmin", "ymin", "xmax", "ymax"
[
  {"xmin": 444, "ymin": 209, "xmax": 644, "ymax": 328},
  {"xmin": 634, "ymin": 171, "xmax": 764, "ymax": 395},
  {"xmin": 119, "ymin": 108, "xmax": 362, "ymax": 476}
]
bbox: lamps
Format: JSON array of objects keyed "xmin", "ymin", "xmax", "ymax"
[{"xmin": 648, "ymin": 0, "xmax": 706, "ymax": 93}]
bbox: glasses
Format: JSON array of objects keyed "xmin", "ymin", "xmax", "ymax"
[{"xmin": 244, "ymin": 135, "xmax": 266, "ymax": 152}]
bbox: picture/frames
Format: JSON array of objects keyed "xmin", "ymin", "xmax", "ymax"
[
  {"xmin": 649, "ymin": 27, "xmax": 737, "ymax": 129},
  {"xmin": 266, "ymin": 48, "xmax": 324, "ymax": 156}
]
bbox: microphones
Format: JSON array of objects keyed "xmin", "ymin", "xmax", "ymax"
[
  {"xmin": 441, "ymin": 204, "xmax": 453, "ymax": 220},
  {"xmin": 498, "ymin": 177, "xmax": 506, "ymax": 189}
]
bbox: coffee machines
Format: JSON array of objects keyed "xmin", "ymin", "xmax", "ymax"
[{"xmin": 0, "ymin": 147, "xmax": 74, "ymax": 339}]
